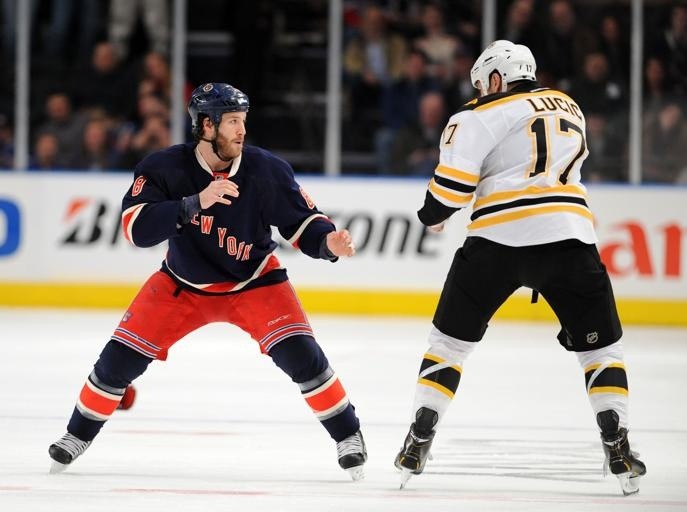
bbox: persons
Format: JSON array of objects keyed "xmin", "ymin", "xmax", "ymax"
[
  {"xmin": 2, "ymin": 0, "xmax": 197, "ymax": 171},
  {"xmin": 45, "ymin": 78, "xmax": 368, "ymax": 470},
  {"xmin": 341, "ymin": 1, "xmax": 481, "ymax": 176},
  {"xmin": 496, "ymin": 1, "xmax": 686, "ymax": 188},
  {"xmin": 394, "ymin": 40, "xmax": 648, "ymax": 483}
]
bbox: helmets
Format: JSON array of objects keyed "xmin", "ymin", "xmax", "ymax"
[
  {"xmin": 188, "ymin": 82, "xmax": 250, "ymax": 136},
  {"xmin": 470, "ymin": 39, "xmax": 537, "ymax": 92}
]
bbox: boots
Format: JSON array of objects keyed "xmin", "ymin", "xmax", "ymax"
[
  {"xmin": 596, "ymin": 409, "xmax": 644, "ymax": 480},
  {"xmin": 337, "ymin": 430, "xmax": 366, "ymax": 468},
  {"xmin": 394, "ymin": 406, "xmax": 439, "ymax": 474},
  {"xmin": 49, "ymin": 431, "xmax": 93, "ymax": 464}
]
